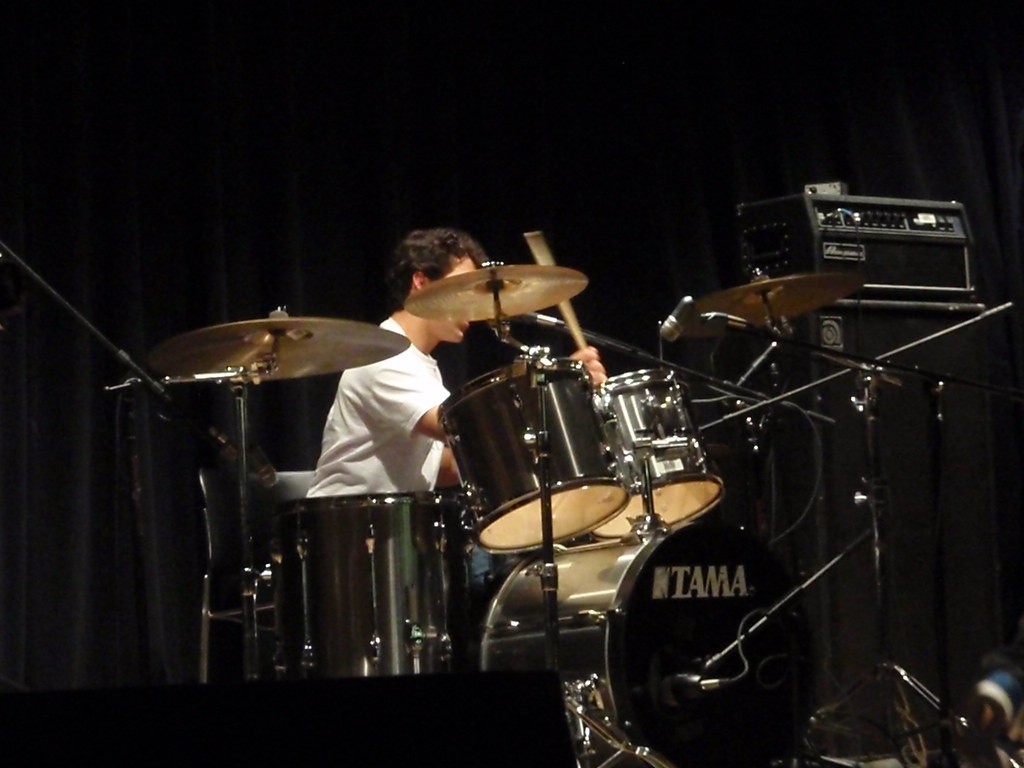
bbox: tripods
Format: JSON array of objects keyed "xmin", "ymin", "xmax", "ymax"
[
  {"xmin": 495, "ymin": 323, "xmax": 673, "ymax": 768},
  {"xmin": 702, "ymin": 310, "xmax": 1022, "ymax": 768}
]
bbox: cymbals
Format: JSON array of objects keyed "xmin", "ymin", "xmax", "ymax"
[
  {"xmin": 152, "ymin": 317, "xmax": 412, "ymax": 381},
  {"xmin": 404, "ymin": 261, "xmax": 588, "ymax": 322},
  {"xmin": 682, "ymin": 271, "xmax": 863, "ymax": 341}
]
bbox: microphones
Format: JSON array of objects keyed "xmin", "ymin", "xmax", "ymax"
[
  {"xmin": 511, "ymin": 311, "xmax": 568, "ymax": 331},
  {"xmin": 661, "ymin": 295, "xmax": 695, "ymax": 343},
  {"xmin": 673, "ymin": 671, "xmax": 721, "ymax": 692},
  {"xmin": 737, "ymin": 339, "xmax": 777, "ymax": 388},
  {"xmin": 210, "ymin": 426, "xmax": 276, "ymax": 490}
]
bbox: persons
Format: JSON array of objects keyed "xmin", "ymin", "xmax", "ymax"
[{"xmin": 302, "ymin": 227, "xmax": 610, "ymax": 611}]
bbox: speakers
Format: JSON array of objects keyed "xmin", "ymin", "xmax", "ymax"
[
  {"xmin": 0, "ymin": 669, "xmax": 576, "ymax": 768},
  {"xmin": 707, "ymin": 297, "xmax": 1001, "ymax": 768}
]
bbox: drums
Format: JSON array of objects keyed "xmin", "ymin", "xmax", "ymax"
[
  {"xmin": 278, "ymin": 489, "xmax": 468, "ymax": 680},
  {"xmin": 589, "ymin": 363, "xmax": 727, "ymax": 543},
  {"xmin": 475, "ymin": 520, "xmax": 818, "ymax": 768},
  {"xmin": 436, "ymin": 358, "xmax": 630, "ymax": 557}
]
